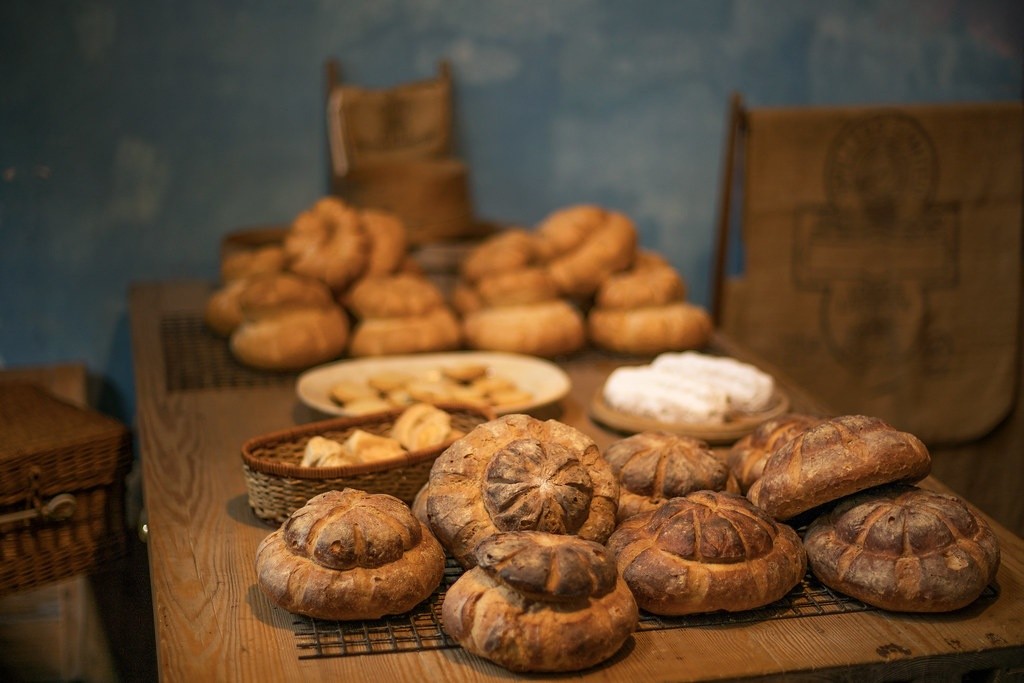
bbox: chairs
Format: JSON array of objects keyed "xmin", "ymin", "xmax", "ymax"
[{"xmin": 714, "ymin": 92, "xmax": 1024, "ymax": 542}]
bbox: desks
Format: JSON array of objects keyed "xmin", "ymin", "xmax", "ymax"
[{"xmin": 125, "ymin": 282, "xmax": 1024, "ymax": 682}]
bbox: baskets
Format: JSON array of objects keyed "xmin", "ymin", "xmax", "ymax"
[
  {"xmin": 240, "ymin": 408, "xmax": 466, "ymax": 528},
  {"xmin": 0, "ymin": 367, "xmax": 134, "ymax": 601}
]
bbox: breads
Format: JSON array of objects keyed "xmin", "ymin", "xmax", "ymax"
[{"xmin": 209, "ymin": 198, "xmax": 1000, "ymax": 672}]
